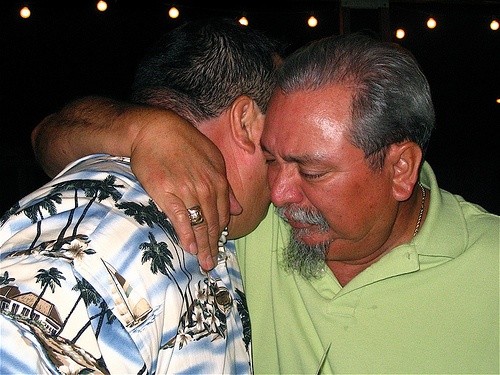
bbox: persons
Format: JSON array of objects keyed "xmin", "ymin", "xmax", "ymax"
[
  {"xmin": 31, "ymin": 34, "xmax": 500, "ymax": 374},
  {"xmin": 0, "ymin": 16, "xmax": 286, "ymax": 374}
]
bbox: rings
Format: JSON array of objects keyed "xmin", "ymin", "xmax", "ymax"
[{"xmin": 187, "ymin": 205, "xmax": 204, "ymax": 226}]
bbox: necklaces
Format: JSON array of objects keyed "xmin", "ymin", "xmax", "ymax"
[{"xmin": 412, "ymin": 180, "xmax": 426, "ymax": 238}]
